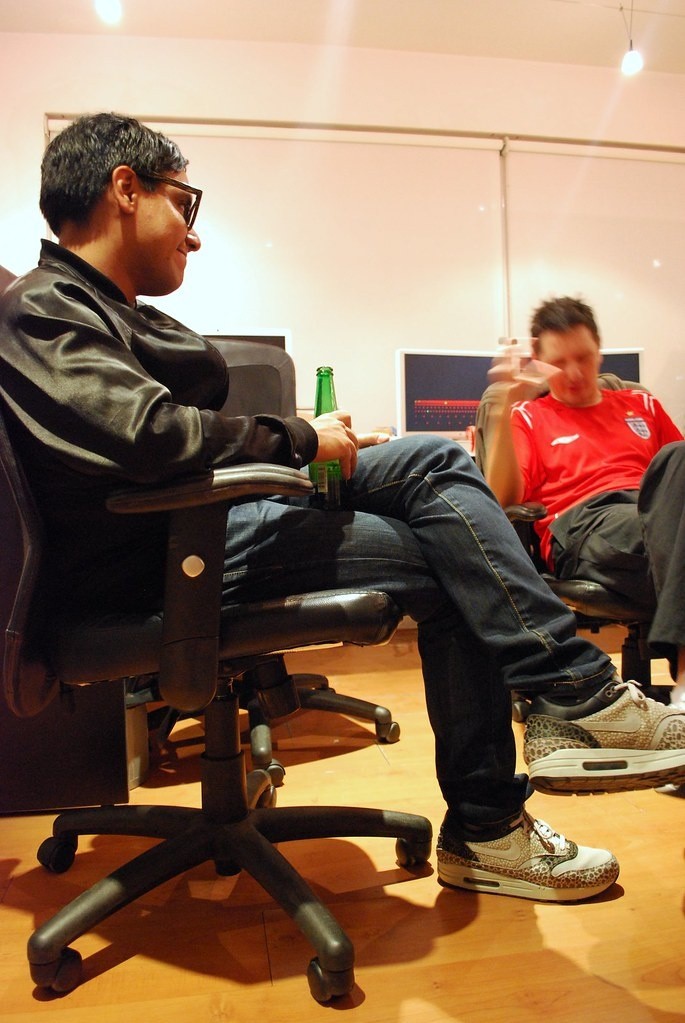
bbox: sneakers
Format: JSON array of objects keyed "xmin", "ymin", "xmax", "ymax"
[
  {"xmin": 437, "ymin": 810, "xmax": 619, "ymax": 903},
  {"xmin": 523, "ymin": 669, "xmax": 685, "ymax": 796}
]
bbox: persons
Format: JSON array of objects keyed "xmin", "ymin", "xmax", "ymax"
[
  {"xmin": 0, "ymin": 111, "xmax": 685, "ymax": 900},
  {"xmin": 483, "ymin": 296, "xmax": 685, "ymax": 796}
]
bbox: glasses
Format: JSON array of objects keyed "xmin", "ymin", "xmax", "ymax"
[{"xmin": 109, "ymin": 166, "xmax": 203, "ymax": 231}]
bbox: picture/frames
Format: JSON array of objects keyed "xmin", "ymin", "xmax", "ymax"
[{"xmin": 395, "ymin": 347, "xmax": 647, "ymax": 441}]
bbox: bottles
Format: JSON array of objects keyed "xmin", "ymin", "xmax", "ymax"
[{"xmin": 309, "ymin": 366, "xmax": 346, "ymax": 510}]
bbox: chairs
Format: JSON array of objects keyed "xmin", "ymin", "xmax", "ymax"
[
  {"xmin": 0, "ymin": 266, "xmax": 433, "ymax": 1007},
  {"xmin": 473, "ymin": 376, "xmax": 675, "ymax": 724}
]
